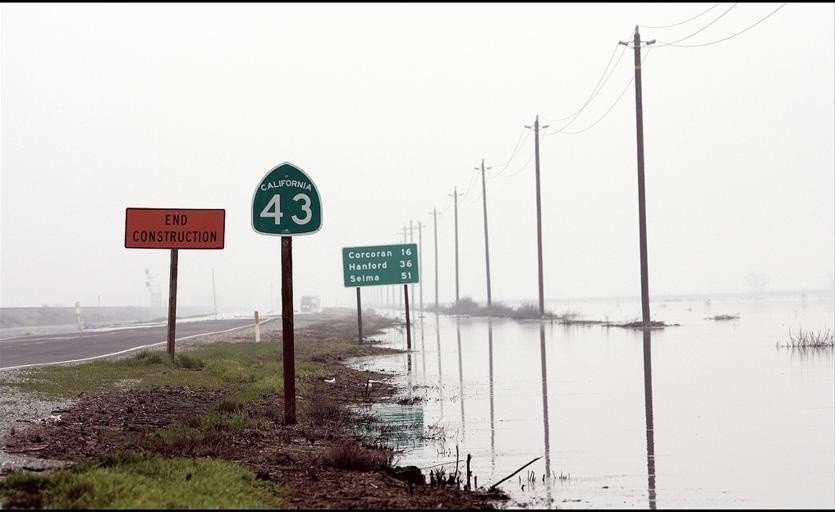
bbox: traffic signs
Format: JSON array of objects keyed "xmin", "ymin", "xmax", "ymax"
[
  {"xmin": 249, "ymin": 161, "xmax": 322, "ymax": 237},
  {"xmin": 342, "ymin": 243, "xmax": 419, "ymax": 288}
]
